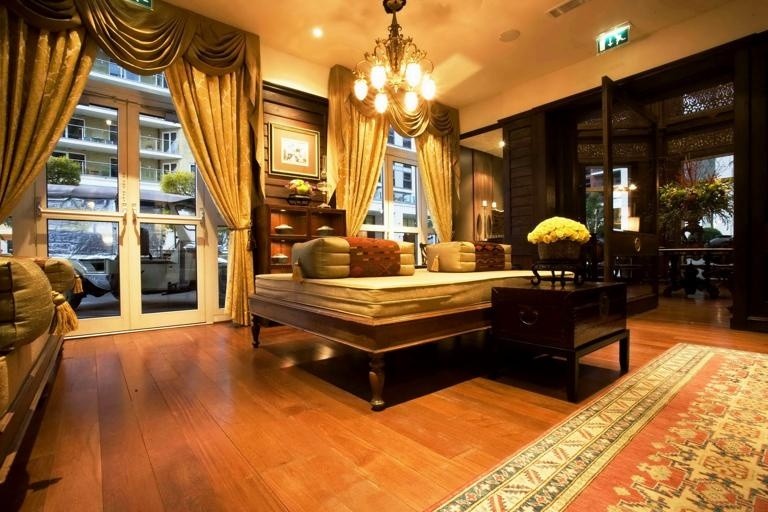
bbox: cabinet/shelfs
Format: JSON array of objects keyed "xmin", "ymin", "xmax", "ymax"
[{"xmin": 263, "ymin": 204, "xmax": 348, "ymax": 272}]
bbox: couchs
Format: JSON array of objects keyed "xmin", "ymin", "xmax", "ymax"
[{"xmin": 1, "ymin": 252, "xmax": 70, "ymax": 445}]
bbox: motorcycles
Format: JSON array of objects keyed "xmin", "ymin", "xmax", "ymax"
[{"xmin": 46, "ymin": 183, "xmax": 229, "ymax": 310}]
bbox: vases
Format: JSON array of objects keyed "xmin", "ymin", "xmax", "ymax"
[{"xmin": 528, "ymin": 236, "xmax": 585, "ymax": 259}]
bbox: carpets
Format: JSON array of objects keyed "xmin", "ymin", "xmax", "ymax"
[{"xmin": 428, "ymin": 341, "xmax": 768, "ymax": 512}]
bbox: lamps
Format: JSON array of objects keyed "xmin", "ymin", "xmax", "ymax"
[{"xmin": 341, "ymin": 0, "xmax": 440, "ymax": 118}]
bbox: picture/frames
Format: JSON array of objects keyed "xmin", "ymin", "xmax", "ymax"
[{"xmin": 268, "ymin": 121, "xmax": 322, "ymax": 181}]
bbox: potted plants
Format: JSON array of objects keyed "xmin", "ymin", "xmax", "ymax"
[{"xmin": 656, "ymin": 177, "xmax": 731, "ymax": 241}]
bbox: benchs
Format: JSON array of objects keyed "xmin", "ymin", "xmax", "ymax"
[{"xmin": 248, "ymin": 265, "xmax": 569, "ymax": 409}]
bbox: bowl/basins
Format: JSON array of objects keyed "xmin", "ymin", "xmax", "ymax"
[
  {"xmin": 276, "ymin": 225, "xmax": 294, "ymax": 235},
  {"xmin": 270, "ymin": 254, "xmax": 289, "ymax": 264}
]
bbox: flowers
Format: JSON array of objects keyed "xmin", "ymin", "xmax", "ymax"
[{"xmin": 526, "ymin": 213, "xmax": 592, "ymax": 245}]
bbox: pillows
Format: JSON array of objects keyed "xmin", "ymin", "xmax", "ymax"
[
  {"xmin": 290, "ymin": 237, "xmax": 413, "ymax": 278},
  {"xmin": 424, "ymin": 241, "xmax": 511, "ymax": 272}
]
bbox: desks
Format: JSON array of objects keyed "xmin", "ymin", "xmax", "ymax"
[
  {"xmin": 656, "ymin": 246, "xmax": 735, "ymax": 296},
  {"xmin": 490, "ymin": 278, "xmax": 631, "ymax": 405}
]
